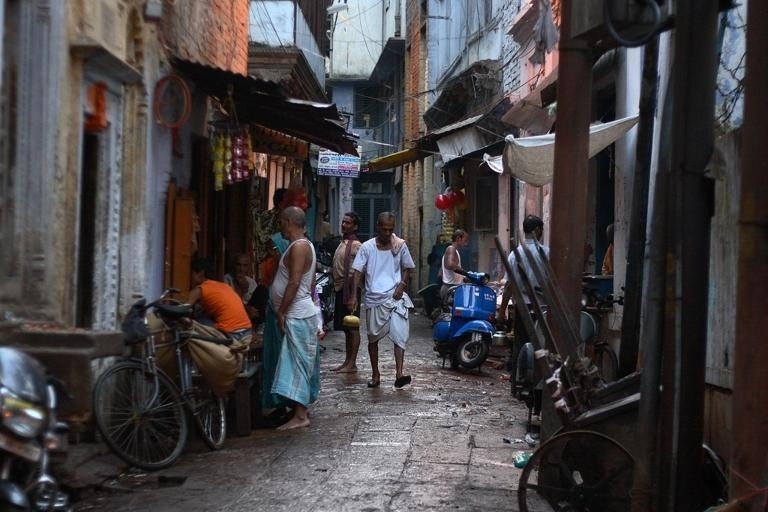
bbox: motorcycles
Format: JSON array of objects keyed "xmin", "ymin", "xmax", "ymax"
[
  {"xmin": 425, "ymin": 269, "xmax": 496, "ymax": 374},
  {"xmin": 316, "ymin": 250, "xmax": 335, "ymax": 325},
  {"xmin": 1, "ymin": 346, "xmax": 69, "ymax": 512}
]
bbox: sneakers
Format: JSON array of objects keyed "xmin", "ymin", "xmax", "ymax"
[
  {"xmin": 368, "ymin": 373, "xmax": 380, "ymax": 387},
  {"xmin": 395, "ymin": 375, "xmax": 411, "ymax": 387},
  {"xmin": 267, "ymin": 408, "xmax": 286, "ymax": 420}
]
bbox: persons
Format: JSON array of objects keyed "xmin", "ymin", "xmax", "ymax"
[
  {"xmin": 347, "ymin": 211, "xmax": 417, "ymax": 388},
  {"xmin": 255, "ymin": 187, "xmax": 296, "ymax": 260},
  {"xmin": 188, "ymin": 259, "xmax": 256, "ymax": 352},
  {"xmin": 601, "ymin": 224, "xmax": 614, "ymax": 277},
  {"xmin": 425, "ymin": 234, "xmax": 448, "ymax": 285},
  {"xmin": 259, "ymin": 204, "xmax": 323, "ymax": 433},
  {"xmin": 437, "ymin": 228, "xmax": 470, "ymax": 313},
  {"xmin": 217, "ymin": 250, "xmax": 273, "ymax": 322},
  {"xmin": 497, "ymin": 213, "xmax": 550, "ymax": 396},
  {"xmin": 328, "ymin": 212, "xmax": 362, "ymax": 374}
]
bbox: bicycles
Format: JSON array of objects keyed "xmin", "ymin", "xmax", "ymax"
[
  {"xmin": 580, "ymin": 285, "xmax": 625, "ymax": 381},
  {"xmin": 93, "ymin": 289, "xmax": 227, "ymax": 469}
]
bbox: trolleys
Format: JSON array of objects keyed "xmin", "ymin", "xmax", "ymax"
[{"xmin": 493, "ymin": 230, "xmax": 641, "ymax": 511}]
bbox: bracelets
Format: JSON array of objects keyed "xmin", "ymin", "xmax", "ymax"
[{"xmin": 400, "ymin": 281, "xmax": 406, "ymax": 286}]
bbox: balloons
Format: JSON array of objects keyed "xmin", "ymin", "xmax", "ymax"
[
  {"xmin": 449, "ymin": 190, "xmax": 465, "ymax": 205},
  {"xmin": 434, "ymin": 194, "xmax": 451, "ymax": 210},
  {"xmin": 455, "ymin": 198, "xmax": 469, "ymax": 210}
]
